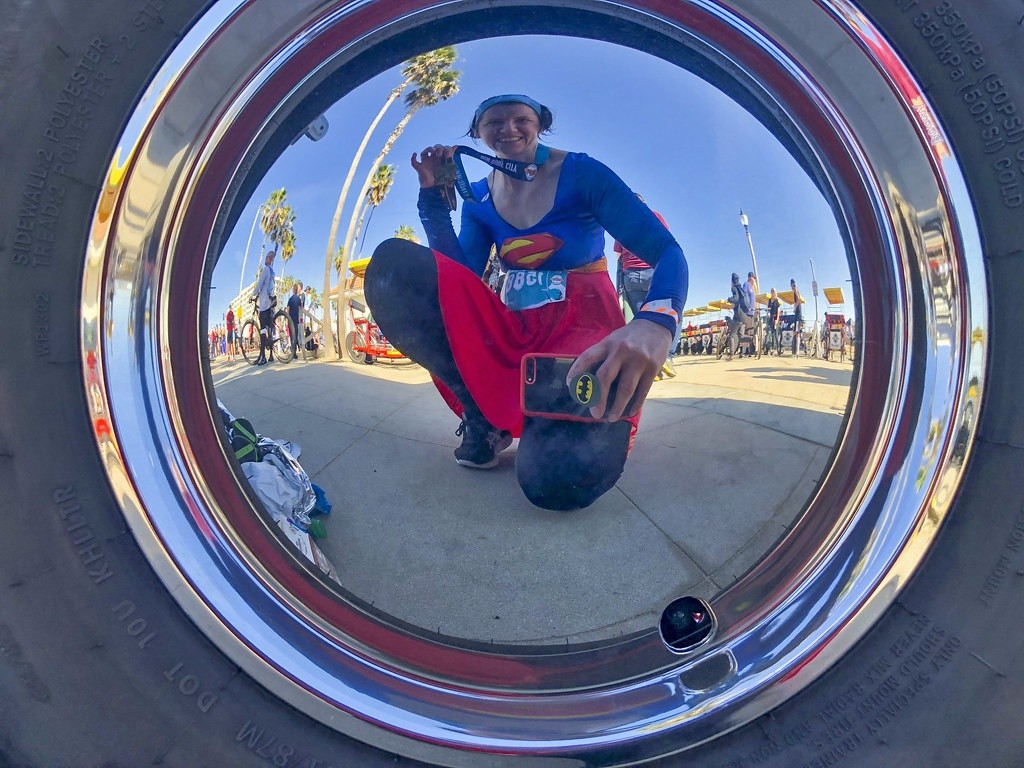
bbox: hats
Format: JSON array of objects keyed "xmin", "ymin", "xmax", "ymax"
[
  {"xmin": 305, "ymin": 327, "xmax": 310, "ymax": 332},
  {"xmin": 298, "ymin": 282, "xmax": 303, "ymax": 289},
  {"xmin": 304, "ymin": 285, "xmax": 311, "ymax": 292},
  {"xmin": 266, "ymin": 251, "xmax": 275, "ymax": 259}
]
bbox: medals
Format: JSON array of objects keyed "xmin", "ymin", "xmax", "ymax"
[{"xmin": 434, "ymin": 164, "xmax": 457, "ymax": 187}]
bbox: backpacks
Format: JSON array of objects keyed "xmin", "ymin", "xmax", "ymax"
[{"xmin": 266, "ymin": 268, "xmax": 278, "ymax": 301}]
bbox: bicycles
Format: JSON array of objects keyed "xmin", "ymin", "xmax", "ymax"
[
  {"xmin": 714, "ymin": 307, "xmax": 772, "ymax": 360},
  {"xmin": 240, "ymin": 296, "xmax": 297, "ymax": 366},
  {"xmin": 761, "ymin": 308, "xmax": 782, "ymax": 355},
  {"xmin": 342, "ymin": 290, "xmax": 409, "ymax": 364}
]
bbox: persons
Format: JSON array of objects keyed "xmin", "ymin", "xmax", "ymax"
[
  {"xmin": 206, "ymin": 251, "xmax": 320, "ymax": 360},
  {"xmin": 364, "ymin": 93, "xmax": 689, "ymax": 511},
  {"xmin": 727, "ymin": 272, "xmax": 801, "ymax": 360},
  {"xmin": 614, "ymin": 194, "xmax": 683, "ymax": 382}
]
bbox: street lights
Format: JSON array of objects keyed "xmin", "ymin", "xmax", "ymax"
[
  {"xmin": 738, "ymin": 206, "xmax": 760, "ymax": 308},
  {"xmin": 808, "ymin": 257, "xmax": 823, "ymax": 359}
]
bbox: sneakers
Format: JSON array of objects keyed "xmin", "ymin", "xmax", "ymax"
[
  {"xmin": 453, "ymin": 406, "xmax": 513, "ymax": 471},
  {"xmin": 267, "ymin": 355, "xmax": 275, "ymax": 362},
  {"xmin": 257, "ymin": 358, "xmax": 267, "ymax": 365}
]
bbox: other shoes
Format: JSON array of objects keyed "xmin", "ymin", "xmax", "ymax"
[
  {"xmin": 227, "ymin": 353, "xmax": 236, "ymax": 363},
  {"xmin": 293, "ymin": 353, "xmax": 298, "ymax": 360}
]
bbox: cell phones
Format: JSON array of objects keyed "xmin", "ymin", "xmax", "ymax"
[{"xmin": 520, "ymin": 354, "xmax": 638, "ymax": 422}]
